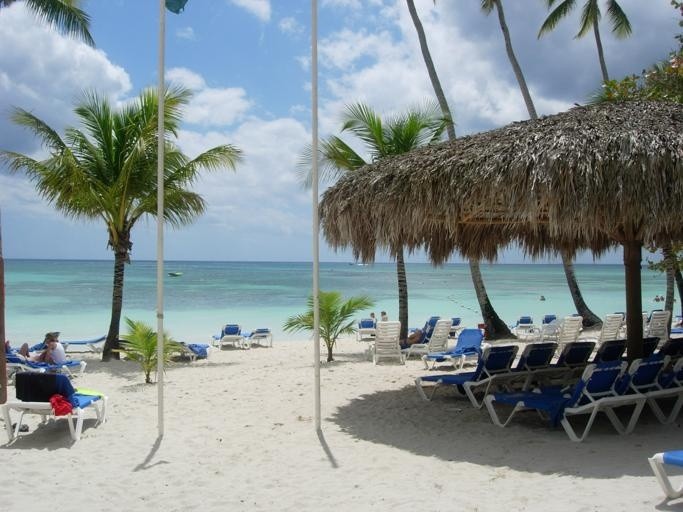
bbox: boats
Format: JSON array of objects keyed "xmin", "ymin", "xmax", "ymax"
[
  {"xmin": 169, "ymin": 271, "xmax": 184, "ymax": 278},
  {"xmin": 348, "ymin": 262, "xmax": 369, "ymax": 266}
]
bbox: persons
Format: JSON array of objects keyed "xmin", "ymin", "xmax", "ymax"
[
  {"xmin": 5, "ymin": 336, "xmax": 67, "ymax": 365},
  {"xmin": 370, "ymin": 311, "xmax": 423, "ymax": 347}
]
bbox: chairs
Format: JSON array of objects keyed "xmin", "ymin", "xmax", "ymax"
[
  {"xmin": 358, "ymin": 310, "xmax": 683, "ymax": 500},
  {"xmin": 3, "ymin": 336, "xmax": 106, "ymax": 442},
  {"xmin": 117, "ymin": 323, "xmax": 273, "ymax": 362}
]
